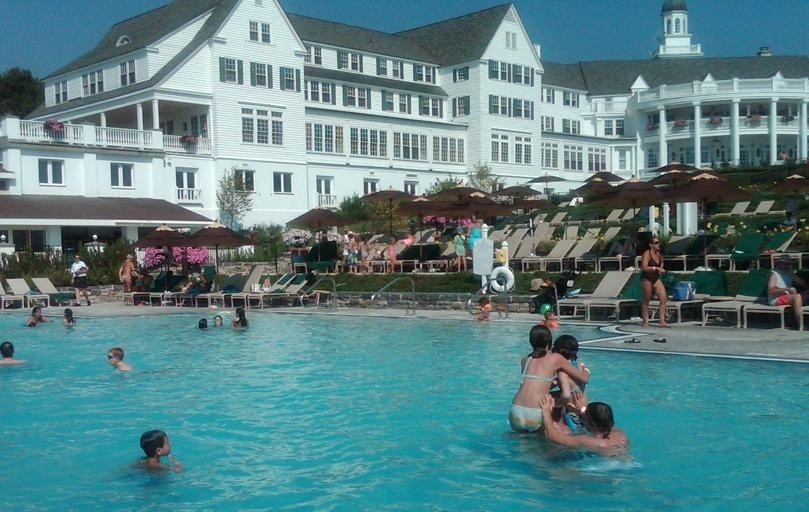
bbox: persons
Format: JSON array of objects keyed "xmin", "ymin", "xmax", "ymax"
[
  {"xmin": 509, "ymin": 325, "xmax": 642, "ymax": 496},
  {"xmin": 449, "ymin": 226, "xmax": 468, "ymax": 272},
  {"xmin": 26, "ymin": 306, "xmax": 76, "ymax": 328},
  {"xmin": 118, "ymin": 254, "xmax": 144, "ymax": 305},
  {"xmin": 0, "ymin": 341, "xmax": 21, "ymax": 364},
  {"xmin": 639, "ymin": 236, "xmax": 671, "ymax": 329},
  {"xmin": 70, "ymin": 255, "xmax": 92, "ymax": 305},
  {"xmin": 340, "ymin": 214, "xmax": 398, "ymax": 275},
  {"xmin": 197, "ymin": 318, "xmax": 207, "ymax": 327},
  {"xmin": 107, "ymin": 348, "xmax": 134, "ymax": 370},
  {"xmin": 213, "ymin": 315, "xmax": 225, "ymax": 327},
  {"xmin": 122, "ymin": 429, "xmax": 185, "ymax": 475},
  {"xmin": 767, "ymin": 254, "xmax": 804, "ymax": 330},
  {"xmin": 232, "ymin": 307, "xmax": 248, "ymax": 328},
  {"xmin": 189, "ymin": 273, "xmax": 207, "ymax": 308},
  {"xmin": 475, "ymin": 298, "xmax": 491, "ymax": 320}
]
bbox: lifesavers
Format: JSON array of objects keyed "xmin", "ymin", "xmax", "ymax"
[{"xmin": 491, "ymin": 268, "xmax": 515, "ymax": 293}]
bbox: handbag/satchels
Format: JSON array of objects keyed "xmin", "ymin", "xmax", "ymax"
[{"xmin": 672, "ymin": 281, "xmax": 697, "ymax": 302}]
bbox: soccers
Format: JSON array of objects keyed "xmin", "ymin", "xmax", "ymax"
[{"xmin": 540, "ymin": 303, "xmax": 551, "ymax": 315}]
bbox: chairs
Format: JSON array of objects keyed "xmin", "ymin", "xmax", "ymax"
[
  {"xmin": 32, "ymin": 278, "xmax": 76, "ymax": 308},
  {"xmin": 556, "ymin": 270, "xmax": 638, "ymax": 323},
  {"xmin": 647, "ymin": 293, "xmax": 808, "ymax": 330},
  {"xmin": 291, "ymin": 200, "xmax": 809, "ymax": 274},
  {"xmin": 0, "ymin": 281, "xmax": 24, "ymax": 310},
  {"xmin": 6, "ymin": 278, "xmax": 50, "ymax": 308},
  {"xmin": 121, "ymin": 263, "xmax": 319, "ymax": 307}
]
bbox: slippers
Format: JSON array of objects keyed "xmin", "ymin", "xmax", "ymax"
[
  {"xmin": 654, "ymin": 337, "xmax": 667, "ymax": 343},
  {"xmin": 625, "ymin": 337, "xmax": 641, "ymax": 343}
]
